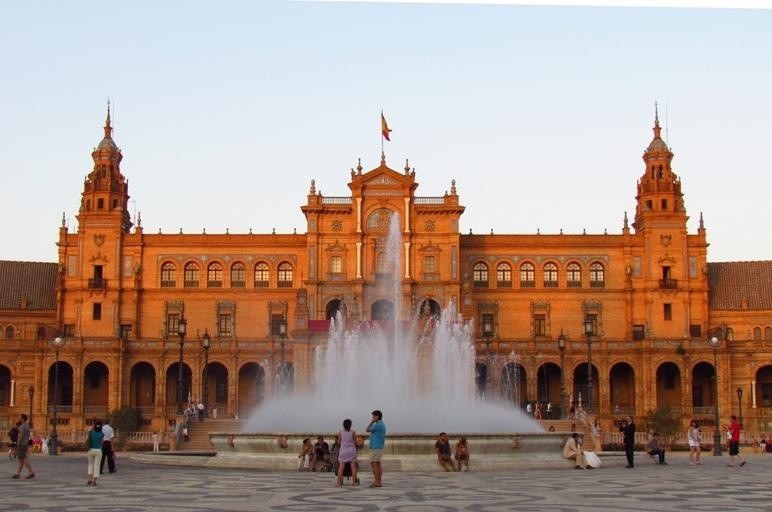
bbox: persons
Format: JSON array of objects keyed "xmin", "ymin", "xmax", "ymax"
[
  {"xmin": 563, "ymin": 432, "xmax": 593, "ymax": 469},
  {"xmin": 365, "ymin": 409, "xmax": 386, "ymax": 487},
  {"xmin": 337, "ymin": 418, "xmax": 360, "ymax": 486},
  {"xmin": 183, "ymin": 423, "xmax": 190, "ymax": 441},
  {"xmin": 435, "ymin": 432, "xmax": 457, "ymax": 472},
  {"xmin": 87, "ymin": 422, "xmax": 104, "ymax": 485},
  {"xmin": 8, "ymin": 413, "xmax": 49, "ymax": 479},
  {"xmin": 646, "ymin": 432, "xmax": 668, "ymax": 465},
  {"xmin": 619, "ymin": 415, "xmax": 636, "ymax": 468},
  {"xmin": 724, "ymin": 414, "xmax": 747, "ymax": 467},
  {"xmin": 102, "ymin": 418, "xmax": 118, "ymax": 473},
  {"xmin": 197, "ymin": 401, "xmax": 204, "ymax": 421},
  {"xmin": 298, "ymin": 436, "xmax": 340, "ymax": 472},
  {"xmin": 454, "ymin": 437, "xmax": 470, "ymax": 472},
  {"xmin": 688, "ymin": 419, "xmax": 703, "ymax": 465},
  {"xmin": 526, "ymin": 400, "xmax": 576, "ymax": 420}
]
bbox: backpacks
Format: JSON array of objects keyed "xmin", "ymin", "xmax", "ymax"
[{"xmin": 7, "ymin": 427, "xmax": 18, "ymax": 442}]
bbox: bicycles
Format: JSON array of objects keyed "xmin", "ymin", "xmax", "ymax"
[{"xmin": 7, "ymin": 440, "xmax": 30, "ymax": 461}]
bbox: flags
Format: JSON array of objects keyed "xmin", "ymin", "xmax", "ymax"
[{"xmin": 382, "ymin": 113, "xmax": 393, "ymax": 142}]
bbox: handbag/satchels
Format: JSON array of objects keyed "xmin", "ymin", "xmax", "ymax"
[
  {"xmin": 334, "ymin": 461, "xmax": 358, "ymax": 480},
  {"xmin": 82, "ymin": 435, "xmax": 90, "ymax": 450},
  {"xmin": 28, "ymin": 440, "xmax": 33, "ymax": 445}
]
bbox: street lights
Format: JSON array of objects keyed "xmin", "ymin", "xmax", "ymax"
[
  {"xmin": 555, "ymin": 327, "xmax": 567, "ymax": 420},
  {"xmin": 481, "ymin": 317, "xmax": 494, "ymax": 395},
  {"xmin": 45, "ymin": 333, "xmax": 67, "ymax": 455},
  {"xmin": 175, "ymin": 311, "xmax": 190, "ymax": 413},
  {"xmin": 735, "ymin": 387, "xmax": 743, "ymax": 430},
  {"xmin": 580, "ymin": 312, "xmax": 594, "ymax": 414},
  {"xmin": 706, "ymin": 334, "xmax": 723, "ymax": 457},
  {"xmin": 198, "ymin": 328, "xmax": 212, "ymax": 419},
  {"xmin": 26, "ymin": 385, "xmax": 35, "ymax": 428},
  {"xmin": 276, "ymin": 317, "xmax": 287, "ymax": 394}
]
